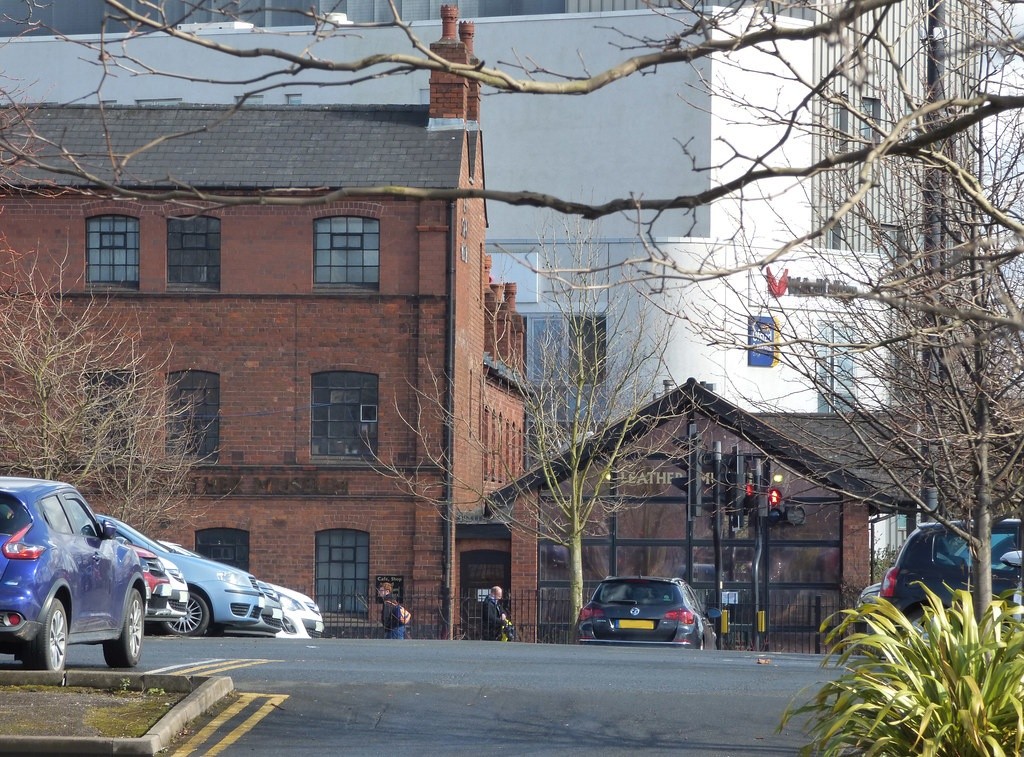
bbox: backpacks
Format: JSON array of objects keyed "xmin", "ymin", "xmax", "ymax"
[{"xmin": 384, "ymin": 599, "xmax": 413, "ymax": 626}]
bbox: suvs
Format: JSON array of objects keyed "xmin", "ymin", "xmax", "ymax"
[
  {"xmin": 877, "ymin": 516, "xmax": 1023, "ymax": 650},
  {"xmin": 574, "ymin": 573, "xmax": 722, "ymax": 651},
  {"xmin": 1, "ymin": 476, "xmax": 146, "ymax": 671}
]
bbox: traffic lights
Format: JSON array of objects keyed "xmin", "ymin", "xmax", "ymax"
[
  {"xmin": 672, "ymin": 422, "xmax": 703, "ymax": 523},
  {"xmin": 765, "ymin": 456, "xmax": 790, "ymax": 520},
  {"xmin": 727, "ymin": 446, "xmax": 752, "ymax": 533}
]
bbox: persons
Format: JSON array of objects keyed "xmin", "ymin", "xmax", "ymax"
[
  {"xmin": 482, "ymin": 586, "xmax": 508, "ymax": 642},
  {"xmin": 380, "ymin": 583, "xmax": 405, "ymax": 640}
]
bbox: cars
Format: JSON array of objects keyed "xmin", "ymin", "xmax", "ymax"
[
  {"xmin": 856, "ymin": 582, "xmax": 881, "ymax": 612},
  {"xmin": 126, "ymin": 541, "xmax": 172, "ymax": 618},
  {"xmin": 157, "ymin": 555, "xmax": 190, "ymax": 623},
  {"xmin": 156, "ymin": 539, "xmax": 325, "ymax": 640},
  {"xmin": 95, "ymin": 513, "xmax": 260, "ymax": 639}
]
